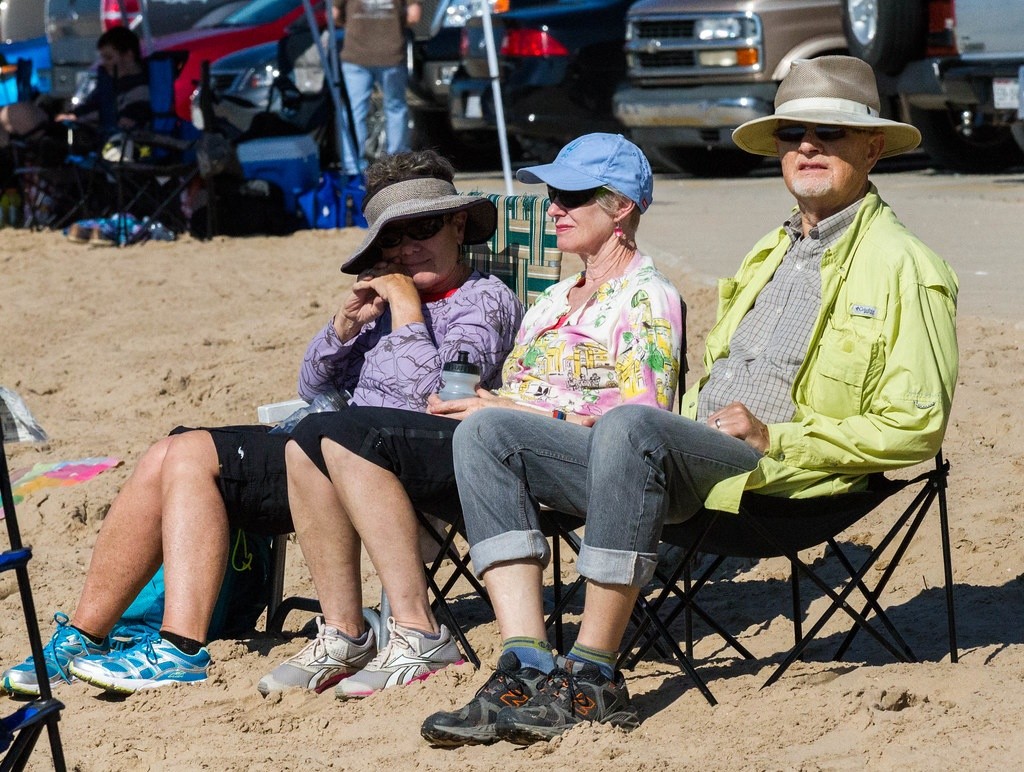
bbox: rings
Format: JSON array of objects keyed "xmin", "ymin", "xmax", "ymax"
[{"xmin": 715, "ymin": 419, "xmax": 721, "ymax": 428}]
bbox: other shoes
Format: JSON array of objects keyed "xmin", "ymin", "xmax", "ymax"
[
  {"xmin": 89, "ymin": 227, "xmax": 115, "ymax": 246},
  {"xmin": 68, "ymin": 225, "xmax": 88, "ymax": 243}
]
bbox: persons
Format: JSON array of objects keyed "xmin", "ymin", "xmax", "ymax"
[
  {"xmin": 331, "ymin": 0, "xmax": 422, "ymax": 174},
  {"xmin": 0, "ymin": 27, "xmax": 150, "ymax": 167},
  {"xmin": 419, "ymin": 56, "xmax": 960, "ymax": 742},
  {"xmin": 257, "ymin": 130, "xmax": 685, "ymax": 698},
  {"xmin": 0, "ymin": 150, "xmax": 524, "ymax": 694}
]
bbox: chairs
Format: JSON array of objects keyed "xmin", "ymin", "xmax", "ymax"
[
  {"xmin": 265, "ymin": 445, "xmax": 959, "ymax": 707},
  {"xmin": 0, "ymin": 50, "xmax": 219, "ymax": 248}
]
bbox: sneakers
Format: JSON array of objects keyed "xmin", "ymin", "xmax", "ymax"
[
  {"xmin": 334, "ymin": 616, "xmax": 465, "ymax": 698},
  {"xmin": 495, "ymin": 654, "xmax": 641, "ymax": 746},
  {"xmin": 0, "ymin": 613, "xmax": 113, "ymax": 697},
  {"xmin": 68, "ymin": 631, "xmax": 218, "ymax": 693},
  {"xmin": 258, "ymin": 616, "xmax": 378, "ymax": 696},
  {"xmin": 420, "ymin": 651, "xmax": 550, "ymax": 747}
]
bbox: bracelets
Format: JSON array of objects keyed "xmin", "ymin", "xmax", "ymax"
[{"xmin": 553, "ymin": 410, "xmax": 566, "ymax": 420}]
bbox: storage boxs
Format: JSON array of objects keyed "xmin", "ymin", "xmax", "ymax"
[{"xmin": 237, "ymin": 134, "xmax": 321, "ymax": 196}]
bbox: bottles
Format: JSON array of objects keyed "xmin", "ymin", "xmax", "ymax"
[{"xmin": 438, "ymin": 350, "xmax": 481, "ymax": 403}]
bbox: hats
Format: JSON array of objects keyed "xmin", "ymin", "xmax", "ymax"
[
  {"xmin": 517, "ymin": 134, "xmax": 654, "ymax": 217},
  {"xmin": 340, "ymin": 176, "xmax": 499, "ymax": 273},
  {"xmin": 736, "ymin": 53, "xmax": 923, "ymax": 160}
]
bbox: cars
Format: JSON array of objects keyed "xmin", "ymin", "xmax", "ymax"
[
  {"xmin": 611, "ymin": 0, "xmax": 1024, "ymax": 180},
  {"xmin": 429, "ymin": 0, "xmax": 637, "ymax": 165},
  {"xmin": 86, "ymin": 0, "xmax": 328, "ymax": 140},
  {"xmin": 190, "ymin": 0, "xmax": 511, "ymax": 150},
  {"xmin": 0, "ymin": 0, "xmax": 246, "ymax": 114}
]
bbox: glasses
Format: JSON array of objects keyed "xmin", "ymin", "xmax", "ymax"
[
  {"xmin": 773, "ymin": 125, "xmax": 843, "ymax": 144},
  {"xmin": 544, "ymin": 182, "xmax": 596, "ymax": 208},
  {"xmin": 377, "ymin": 213, "xmax": 452, "ymax": 242}
]
